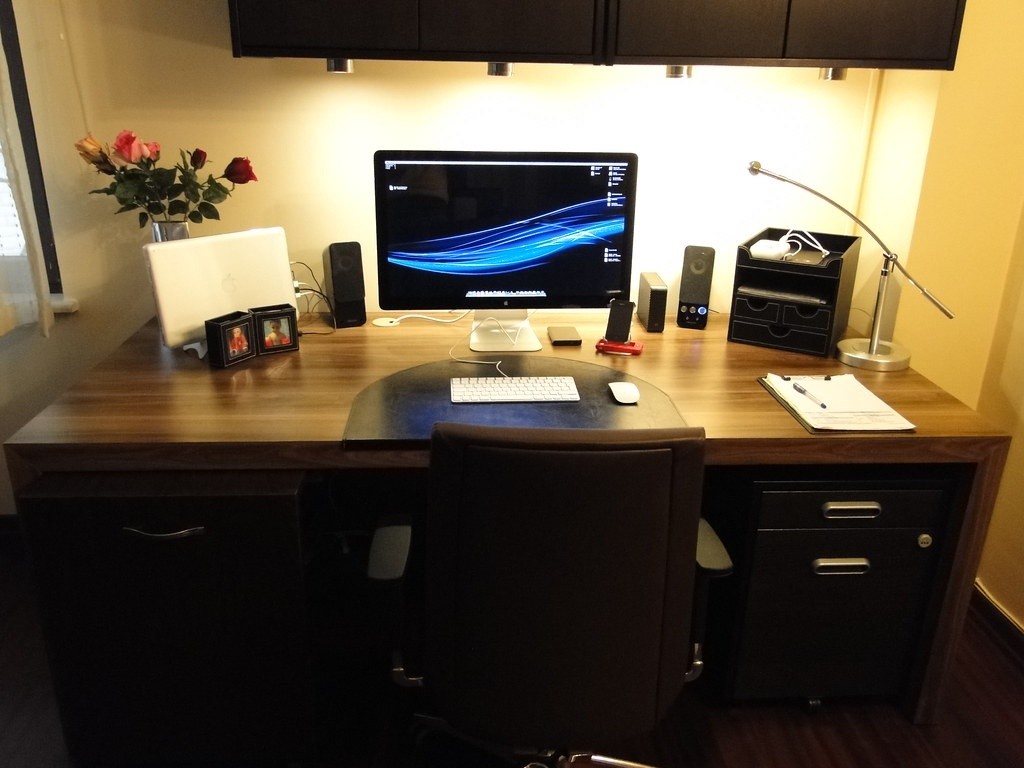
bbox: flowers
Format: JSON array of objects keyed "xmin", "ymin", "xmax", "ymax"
[{"xmin": 77, "ymin": 130, "xmax": 260, "ymax": 222}]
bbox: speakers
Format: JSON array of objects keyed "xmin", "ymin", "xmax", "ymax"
[
  {"xmin": 322, "ymin": 241, "xmax": 366, "ymax": 329},
  {"xmin": 676, "ymin": 245, "xmax": 716, "ymax": 329}
]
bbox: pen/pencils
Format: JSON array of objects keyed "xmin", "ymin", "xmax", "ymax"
[{"xmin": 793, "ymin": 381, "xmax": 826, "ymax": 409}]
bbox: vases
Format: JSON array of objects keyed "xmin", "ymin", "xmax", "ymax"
[{"xmin": 153, "ymin": 224, "xmax": 190, "ymax": 242}]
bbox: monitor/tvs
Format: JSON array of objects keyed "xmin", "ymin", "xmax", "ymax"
[{"xmin": 374, "ymin": 150, "xmax": 638, "ymax": 351}]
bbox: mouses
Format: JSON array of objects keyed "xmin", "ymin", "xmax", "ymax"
[{"xmin": 608, "ymin": 381, "xmax": 640, "ymax": 404}]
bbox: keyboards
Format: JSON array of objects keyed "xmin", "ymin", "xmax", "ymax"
[{"xmin": 451, "ymin": 376, "xmax": 581, "ymax": 402}]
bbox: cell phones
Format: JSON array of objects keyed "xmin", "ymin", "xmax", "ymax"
[{"xmin": 605, "ymin": 300, "xmax": 634, "ymax": 342}]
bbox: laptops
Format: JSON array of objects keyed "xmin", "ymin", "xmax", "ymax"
[{"xmin": 143, "ymin": 226, "xmax": 299, "ymax": 349}]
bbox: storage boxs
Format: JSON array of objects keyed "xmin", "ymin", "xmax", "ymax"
[
  {"xmin": 248, "ymin": 304, "xmax": 299, "ymax": 355},
  {"xmin": 205, "ymin": 311, "xmax": 256, "ymax": 370},
  {"xmin": 725, "ymin": 227, "xmax": 860, "ymax": 359}
]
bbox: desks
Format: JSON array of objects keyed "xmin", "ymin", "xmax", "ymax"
[{"xmin": 0, "ymin": 312, "xmax": 1013, "ymax": 768}]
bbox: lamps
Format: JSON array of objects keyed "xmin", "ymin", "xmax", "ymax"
[{"xmin": 749, "ymin": 161, "xmax": 952, "ymax": 372}]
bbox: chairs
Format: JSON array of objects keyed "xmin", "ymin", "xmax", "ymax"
[{"xmin": 368, "ymin": 422, "xmax": 733, "ymax": 768}]
